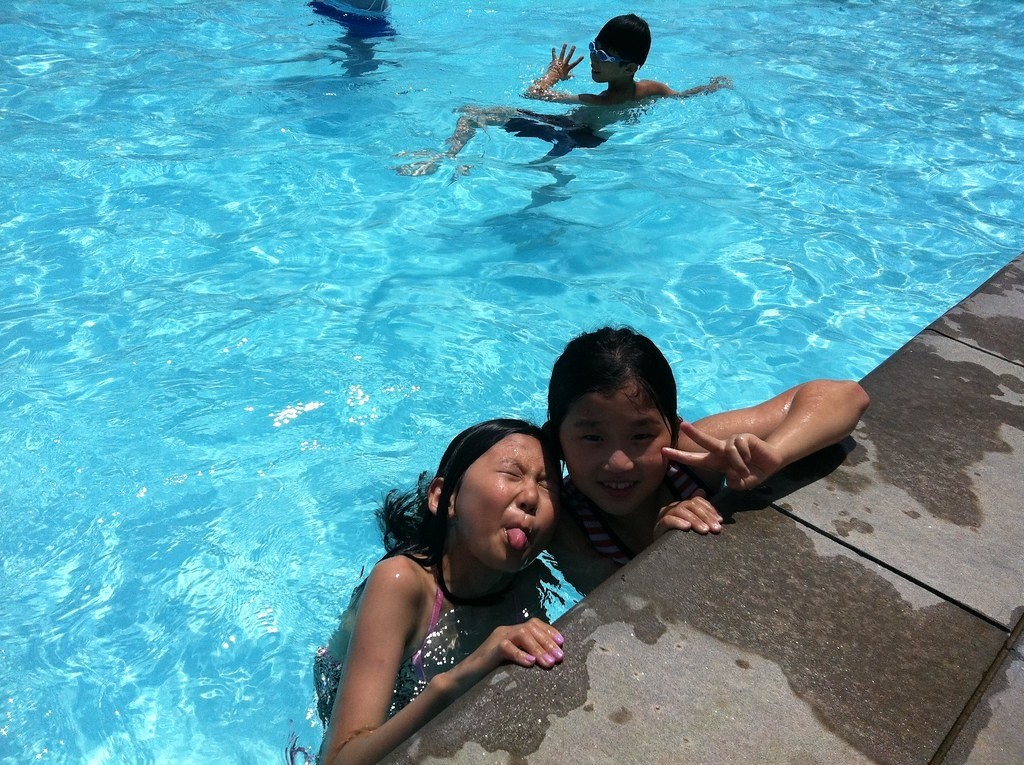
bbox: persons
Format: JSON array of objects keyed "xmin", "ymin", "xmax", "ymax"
[
  {"xmin": 547, "ymin": 329, "xmax": 870, "ymax": 595},
  {"xmin": 314, "ymin": 419, "xmax": 564, "ymax": 765},
  {"xmin": 527, "ymin": 14, "xmax": 692, "ymax": 103}
]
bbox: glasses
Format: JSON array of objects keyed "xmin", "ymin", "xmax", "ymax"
[{"xmin": 589, "ymin": 40, "xmax": 633, "ymax": 63}]
